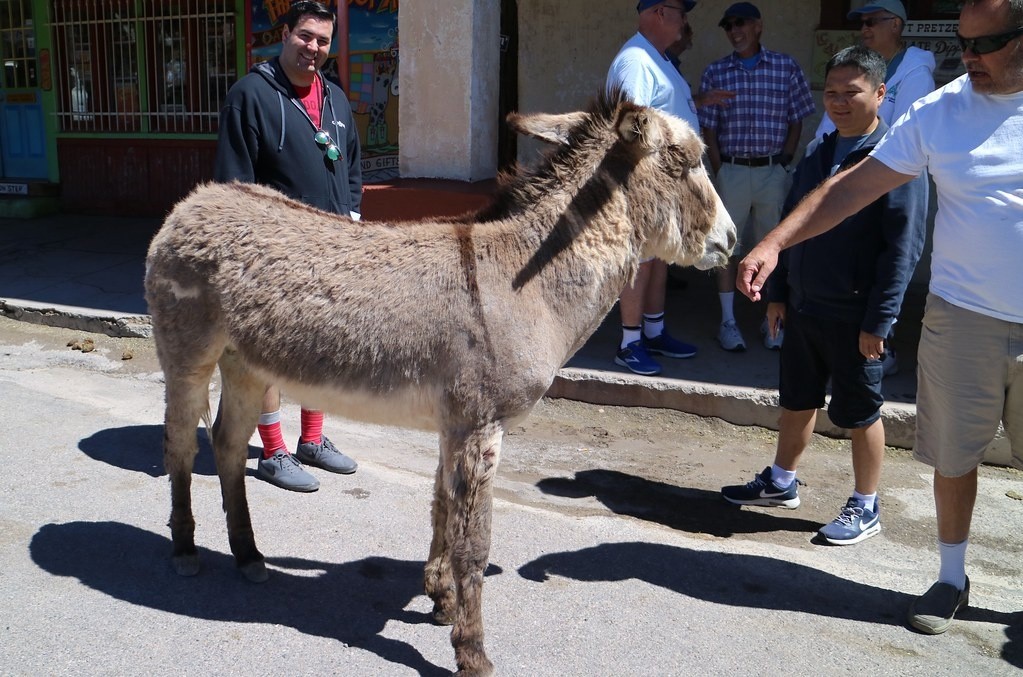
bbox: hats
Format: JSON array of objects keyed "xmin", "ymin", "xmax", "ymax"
[
  {"xmin": 848, "ymin": 0, "xmax": 907, "ymax": 25},
  {"xmin": 637, "ymin": 0, "xmax": 698, "ymax": 14},
  {"xmin": 718, "ymin": 3, "xmax": 761, "ymax": 27}
]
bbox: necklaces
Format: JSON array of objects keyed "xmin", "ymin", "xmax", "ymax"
[{"xmin": 885, "ymin": 44, "xmax": 902, "ymax": 66}]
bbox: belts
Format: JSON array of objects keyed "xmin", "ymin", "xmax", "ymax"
[{"xmin": 720, "ymin": 153, "xmax": 781, "ymax": 168}]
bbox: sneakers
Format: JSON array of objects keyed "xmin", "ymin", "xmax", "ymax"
[
  {"xmin": 258, "ymin": 449, "xmax": 320, "ymax": 491},
  {"xmin": 817, "ymin": 497, "xmax": 879, "ymax": 546},
  {"xmin": 722, "ymin": 466, "xmax": 801, "ymax": 509},
  {"xmin": 908, "ymin": 574, "xmax": 970, "ymax": 635},
  {"xmin": 717, "ymin": 319, "xmax": 746, "ymax": 352},
  {"xmin": 760, "ymin": 316, "xmax": 784, "ymax": 349},
  {"xmin": 614, "ymin": 340, "xmax": 663, "ymax": 375},
  {"xmin": 641, "ymin": 328, "xmax": 697, "ymax": 358},
  {"xmin": 296, "ymin": 435, "xmax": 358, "ymax": 474}
]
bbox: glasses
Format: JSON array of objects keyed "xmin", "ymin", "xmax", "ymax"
[
  {"xmin": 314, "ymin": 128, "xmax": 344, "ymax": 161},
  {"xmin": 857, "ymin": 17, "xmax": 896, "ymax": 30},
  {"xmin": 654, "ymin": 5, "xmax": 686, "ymax": 18},
  {"xmin": 956, "ymin": 27, "xmax": 1023, "ymax": 55},
  {"xmin": 723, "ymin": 19, "xmax": 754, "ymax": 32}
]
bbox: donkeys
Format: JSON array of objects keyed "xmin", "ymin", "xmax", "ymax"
[{"xmin": 142, "ymin": 72, "xmax": 739, "ymax": 677}]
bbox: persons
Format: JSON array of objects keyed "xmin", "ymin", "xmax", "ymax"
[
  {"xmin": 696, "ymin": 2, "xmax": 817, "ymax": 352},
  {"xmin": 606, "ymin": 0, "xmax": 737, "ymax": 374},
  {"xmin": 212, "ymin": 0, "xmax": 363, "ymax": 494},
  {"xmin": 807, "ymin": 0, "xmax": 936, "ymax": 378},
  {"xmin": 721, "ymin": 45, "xmax": 927, "ymax": 544},
  {"xmin": 737, "ymin": 0, "xmax": 1023, "ymax": 635}
]
